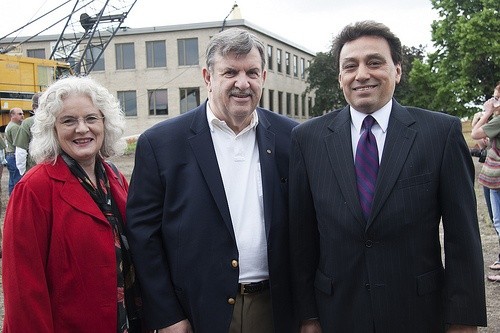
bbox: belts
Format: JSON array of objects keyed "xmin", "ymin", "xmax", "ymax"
[{"xmin": 238, "ymin": 280, "xmax": 268, "ymax": 295}]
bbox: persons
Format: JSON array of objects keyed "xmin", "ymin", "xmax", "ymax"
[
  {"xmin": 2, "ymin": 76, "xmax": 129, "ymax": 333},
  {"xmin": 12, "ymin": 93, "xmax": 42, "ymax": 175},
  {"xmin": 472, "ymin": 85, "xmax": 500, "ymax": 281},
  {"xmin": 288, "ymin": 25, "xmax": 488, "ymax": 333},
  {"xmin": 0, "ymin": 137, "xmax": 6, "ymax": 180},
  {"xmin": 4, "ymin": 106, "xmax": 24, "ymax": 192},
  {"xmin": 126, "ymin": 28, "xmax": 300, "ymax": 333}
]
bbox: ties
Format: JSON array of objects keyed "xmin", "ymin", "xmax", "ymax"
[{"xmin": 354, "ymin": 115, "xmax": 380, "ymax": 224}]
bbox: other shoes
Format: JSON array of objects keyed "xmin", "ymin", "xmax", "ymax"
[
  {"xmin": 490, "ymin": 260, "xmax": 500, "ymax": 269},
  {"xmin": 487, "ymin": 273, "xmax": 500, "ymax": 282}
]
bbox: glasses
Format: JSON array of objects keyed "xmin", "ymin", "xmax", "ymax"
[
  {"xmin": 56, "ymin": 115, "xmax": 105, "ymax": 129},
  {"xmin": 16, "ymin": 112, "xmax": 25, "ymax": 116}
]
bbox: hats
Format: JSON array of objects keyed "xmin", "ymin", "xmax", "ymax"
[{"xmin": 32, "ymin": 93, "xmax": 43, "ymax": 104}]
